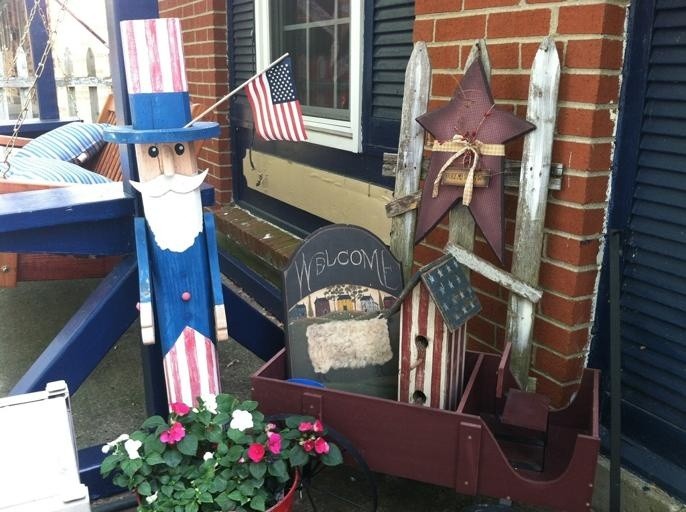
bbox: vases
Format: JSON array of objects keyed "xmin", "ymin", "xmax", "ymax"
[{"xmin": 136, "ymin": 463, "xmax": 299, "ymax": 512}]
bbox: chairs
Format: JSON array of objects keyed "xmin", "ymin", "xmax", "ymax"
[{"xmin": 0, "ymin": 91, "xmax": 214, "ymax": 288}]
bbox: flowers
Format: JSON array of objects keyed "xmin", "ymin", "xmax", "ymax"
[{"xmin": 100, "ymin": 394, "xmax": 344, "ymax": 512}]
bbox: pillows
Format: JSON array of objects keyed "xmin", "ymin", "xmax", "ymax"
[
  {"xmin": 15, "ymin": 120, "xmax": 117, "ymax": 163},
  {"xmin": 0, "ymin": 157, "xmax": 114, "ymax": 186}
]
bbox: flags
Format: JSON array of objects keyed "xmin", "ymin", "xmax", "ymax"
[{"xmin": 246, "ymin": 57, "xmax": 308, "ymax": 142}]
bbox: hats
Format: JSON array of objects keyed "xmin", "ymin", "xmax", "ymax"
[{"xmin": 102, "ymin": 18, "xmax": 220, "ymax": 144}]
